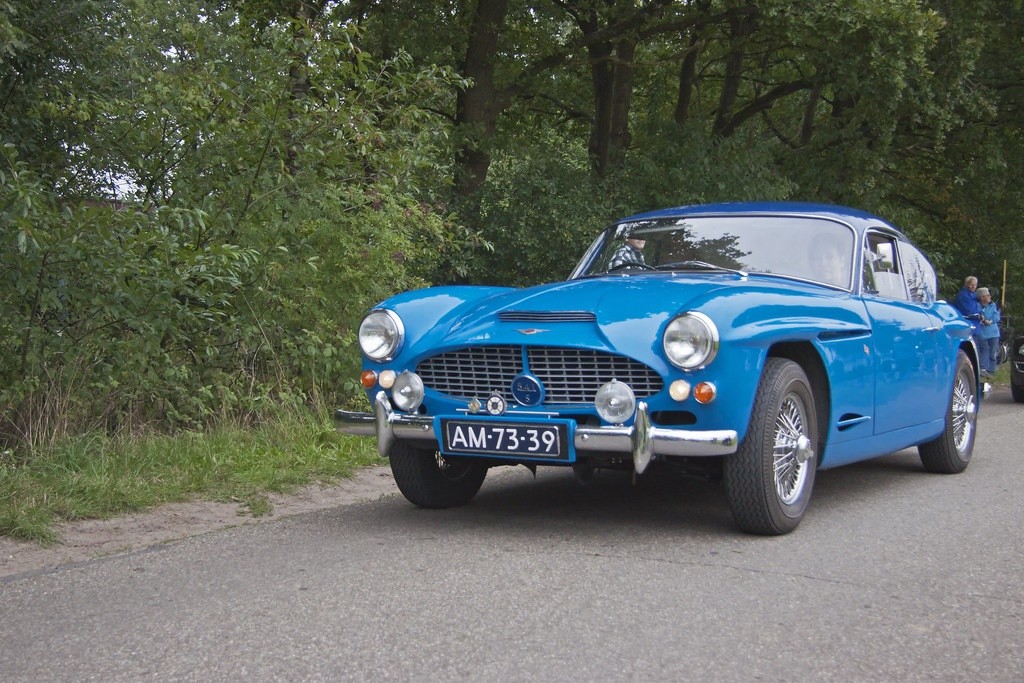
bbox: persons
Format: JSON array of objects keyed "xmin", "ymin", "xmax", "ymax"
[
  {"xmin": 608, "ymin": 238, "xmax": 646, "ymax": 271},
  {"xmin": 955, "ymin": 276, "xmax": 1001, "ymax": 378}
]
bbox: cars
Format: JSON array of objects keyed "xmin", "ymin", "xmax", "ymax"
[
  {"xmin": 1011, "ymin": 332, "xmax": 1024, "ymax": 404},
  {"xmin": 331, "ymin": 198, "xmax": 982, "ymax": 538}
]
